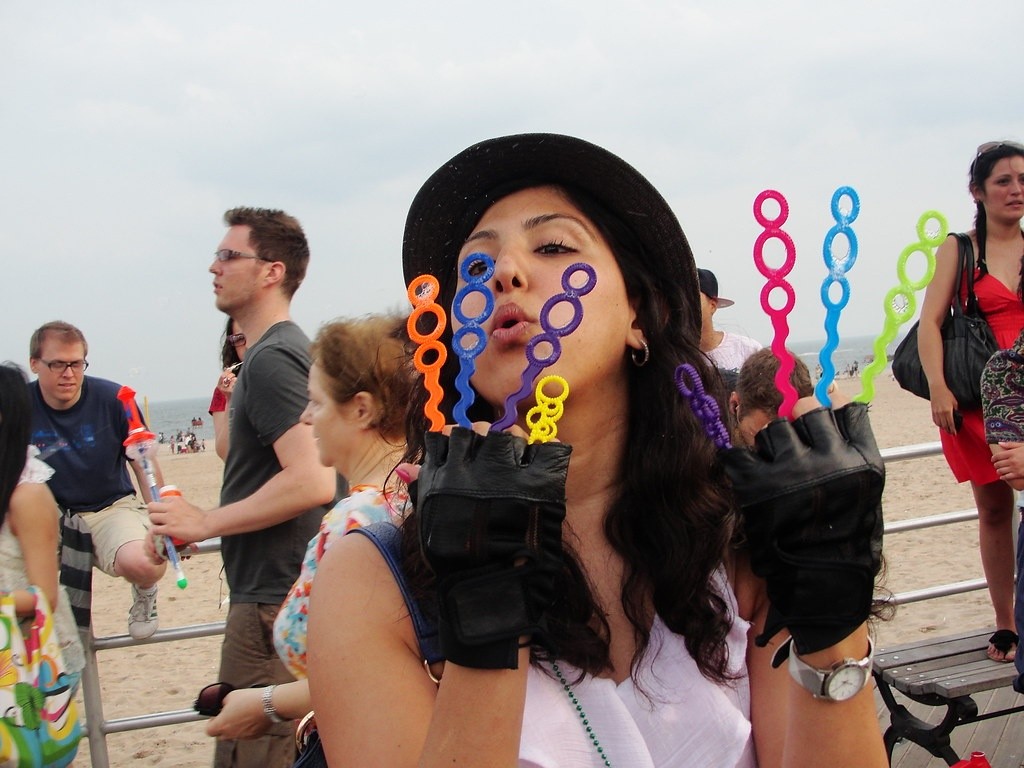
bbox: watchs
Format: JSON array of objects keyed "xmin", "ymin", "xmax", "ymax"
[{"xmin": 770, "ymin": 635, "xmax": 874, "ymax": 702}]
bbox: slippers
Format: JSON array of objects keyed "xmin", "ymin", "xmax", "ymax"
[{"xmin": 985, "ymin": 630, "xmax": 1018, "ymax": 662}]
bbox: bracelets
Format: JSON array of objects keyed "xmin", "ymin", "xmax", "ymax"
[{"xmin": 262, "ymin": 685, "xmax": 290, "ymax": 723}]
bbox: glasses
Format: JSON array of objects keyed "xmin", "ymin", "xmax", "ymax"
[
  {"xmin": 194, "ymin": 683, "xmax": 291, "ymax": 737},
  {"xmin": 974, "ymin": 141, "xmax": 1024, "ymax": 178},
  {"xmin": 216, "ymin": 250, "xmax": 275, "ymax": 262},
  {"xmin": 37, "ymin": 358, "xmax": 89, "ymax": 374},
  {"xmin": 226, "ymin": 333, "xmax": 247, "ymax": 347}
]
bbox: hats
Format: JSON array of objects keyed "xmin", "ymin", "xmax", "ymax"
[
  {"xmin": 403, "ymin": 133, "xmax": 701, "ymax": 350},
  {"xmin": 698, "ymin": 268, "xmax": 734, "ymax": 309}
]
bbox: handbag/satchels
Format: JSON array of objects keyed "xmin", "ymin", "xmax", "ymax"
[{"xmin": 892, "ymin": 234, "xmax": 1000, "ymax": 407}]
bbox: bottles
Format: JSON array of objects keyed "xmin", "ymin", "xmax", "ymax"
[{"xmin": 158, "ymin": 484, "xmax": 184, "ymax": 547}]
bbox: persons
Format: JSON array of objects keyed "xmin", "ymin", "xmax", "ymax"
[
  {"xmin": 980, "ymin": 329, "xmax": 1024, "ymax": 696},
  {"xmin": 147, "ymin": 203, "xmax": 350, "ymax": 768},
  {"xmin": 0, "ymin": 364, "xmax": 80, "ymax": 768},
  {"xmin": 209, "ymin": 317, "xmax": 247, "ymax": 462},
  {"xmin": 917, "ymin": 139, "xmax": 1024, "ymax": 662},
  {"xmin": 719, "ymin": 345, "xmax": 814, "ymax": 450},
  {"xmin": 159, "ymin": 432, "xmax": 164, "ymax": 444},
  {"xmin": 697, "ymin": 267, "xmax": 764, "ymax": 374},
  {"xmin": 22, "ymin": 320, "xmax": 199, "ymax": 639},
  {"xmin": 207, "ymin": 317, "xmax": 422, "ymax": 744},
  {"xmin": 169, "ymin": 427, "xmax": 206, "ymax": 454},
  {"xmin": 305, "ymin": 133, "xmax": 888, "ymax": 768},
  {"xmin": 191, "ymin": 416, "xmax": 201, "ymax": 423}
]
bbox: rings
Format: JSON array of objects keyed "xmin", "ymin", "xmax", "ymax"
[{"xmin": 223, "ymin": 378, "xmax": 231, "ymax": 386}]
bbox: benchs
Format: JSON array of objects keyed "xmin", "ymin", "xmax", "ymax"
[{"xmin": 868, "ymin": 625, "xmax": 1024, "ymax": 768}]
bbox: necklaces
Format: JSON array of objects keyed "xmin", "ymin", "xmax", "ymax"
[{"xmin": 548, "ymin": 656, "xmax": 611, "ymax": 768}]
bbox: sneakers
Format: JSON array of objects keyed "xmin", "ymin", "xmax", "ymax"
[{"xmin": 127, "ymin": 582, "xmax": 159, "ymax": 640}]
